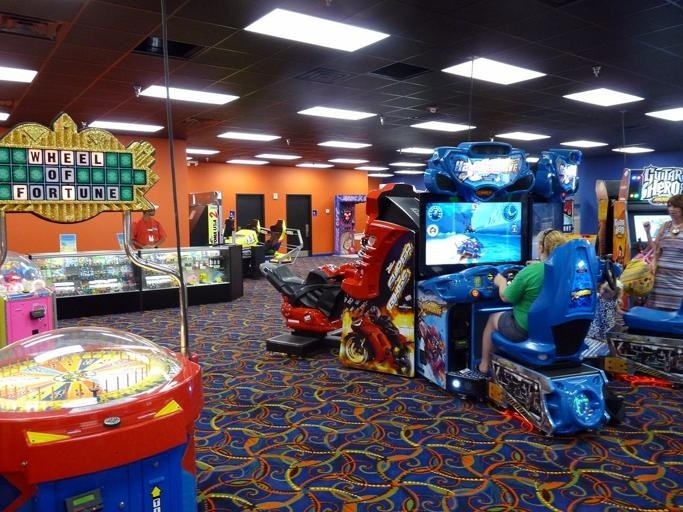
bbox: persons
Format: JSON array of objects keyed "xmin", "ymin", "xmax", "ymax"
[
  {"xmin": 130, "ymin": 210, "xmax": 167, "ymax": 249},
  {"xmin": 641, "ymin": 194, "xmax": 683, "ymax": 311},
  {"xmin": 471, "ymin": 227, "xmax": 566, "ymax": 377},
  {"xmin": 586, "ymin": 278, "xmax": 627, "ymax": 341}
]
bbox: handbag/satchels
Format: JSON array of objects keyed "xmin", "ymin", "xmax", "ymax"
[{"xmin": 619, "ymin": 251, "xmax": 657, "ymax": 298}]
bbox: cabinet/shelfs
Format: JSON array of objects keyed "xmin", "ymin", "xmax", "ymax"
[{"xmin": 28, "ymin": 245, "xmax": 241, "ymax": 319}]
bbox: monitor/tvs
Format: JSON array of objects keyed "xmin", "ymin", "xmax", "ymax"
[
  {"xmin": 632, "ymin": 214, "xmax": 673, "ymax": 243},
  {"xmin": 419, "ymin": 195, "xmax": 528, "ymax": 267},
  {"xmin": 528, "ymin": 200, "xmax": 564, "ymax": 261}
]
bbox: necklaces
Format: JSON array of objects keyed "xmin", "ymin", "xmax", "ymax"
[{"xmin": 669, "ymin": 220, "xmax": 682, "ymax": 234}]
